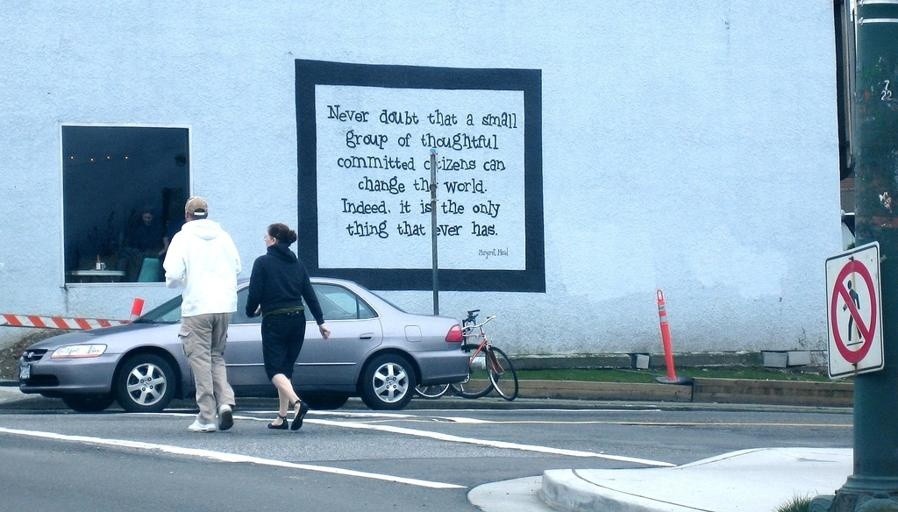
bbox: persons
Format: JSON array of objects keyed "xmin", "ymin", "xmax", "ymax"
[
  {"xmin": 163, "ymin": 197, "xmax": 242, "ymax": 432},
  {"xmin": 246, "ymin": 224, "xmax": 331, "ymax": 430},
  {"xmin": 133, "ymin": 209, "xmax": 169, "ymax": 255}
]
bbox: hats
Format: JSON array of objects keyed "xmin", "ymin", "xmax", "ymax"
[{"xmin": 184, "ymin": 196, "xmax": 208, "ymax": 216}]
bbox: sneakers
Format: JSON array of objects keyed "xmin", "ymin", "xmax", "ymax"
[
  {"xmin": 291, "ymin": 401, "xmax": 309, "ymax": 430},
  {"xmin": 188, "ymin": 418, "xmax": 216, "ymax": 433},
  {"xmin": 220, "ymin": 405, "xmax": 234, "ymax": 430},
  {"xmin": 267, "ymin": 414, "xmax": 289, "ymax": 430}
]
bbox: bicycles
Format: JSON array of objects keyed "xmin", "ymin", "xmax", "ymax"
[{"xmin": 413, "ymin": 305, "xmax": 520, "ymax": 402}]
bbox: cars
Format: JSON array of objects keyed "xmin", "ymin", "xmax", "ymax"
[{"xmin": 12, "ymin": 274, "xmax": 471, "ymax": 409}]
